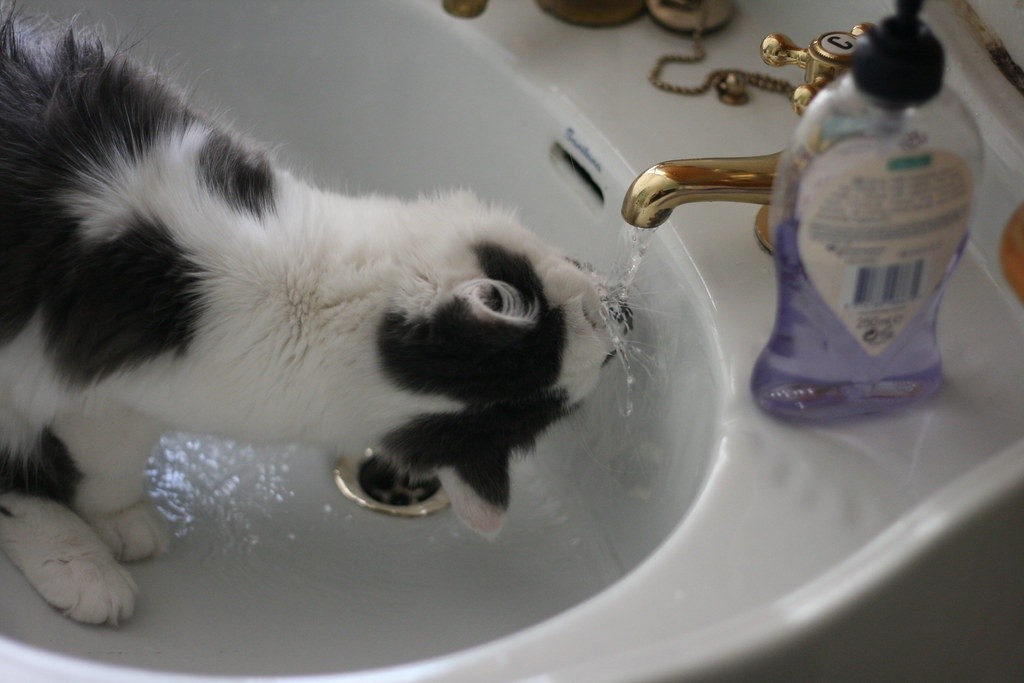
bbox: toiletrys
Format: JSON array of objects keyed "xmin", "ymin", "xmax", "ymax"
[{"xmin": 748, "ymin": 0, "xmax": 988, "ymax": 424}]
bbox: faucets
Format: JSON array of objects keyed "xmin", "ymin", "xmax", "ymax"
[{"xmin": 618, "ymin": 52, "xmax": 846, "ymax": 242}]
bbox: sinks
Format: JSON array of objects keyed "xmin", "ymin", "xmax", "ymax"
[{"xmin": 0, "ymin": 1, "xmax": 735, "ymax": 683}]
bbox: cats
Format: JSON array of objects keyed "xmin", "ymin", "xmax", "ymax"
[{"xmin": 0, "ymin": 2, "xmax": 635, "ymax": 628}]
